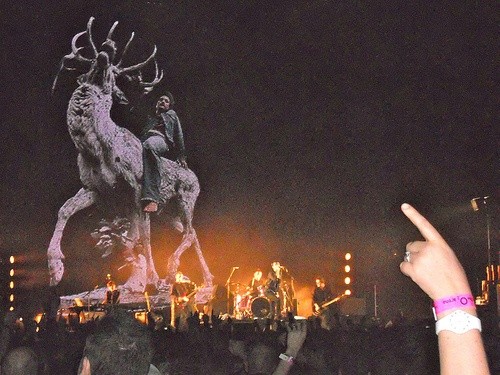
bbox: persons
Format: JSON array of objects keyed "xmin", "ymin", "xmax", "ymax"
[
  {"xmin": 170, "ymin": 270, "xmax": 196, "ymax": 317},
  {"xmin": 121, "ymin": 238, "xmax": 147, "ymax": 296},
  {"xmin": 245, "ymin": 268, "xmax": 266, "ymax": 298},
  {"xmin": 137, "ymin": 89, "xmax": 187, "ymax": 214},
  {"xmin": 310, "ymin": 275, "xmax": 337, "ymax": 318},
  {"xmin": 398, "ymin": 195, "xmax": 494, "ymax": 375},
  {"xmin": 260, "ymin": 259, "xmax": 283, "ymax": 318},
  {"xmin": 1, "ymin": 298, "xmax": 498, "ymax": 375}
]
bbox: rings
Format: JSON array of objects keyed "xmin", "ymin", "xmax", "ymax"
[{"xmin": 403, "ymin": 250, "xmax": 411, "ymax": 262}]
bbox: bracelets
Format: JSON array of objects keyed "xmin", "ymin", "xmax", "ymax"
[{"xmin": 428, "ymin": 292, "xmax": 477, "ymax": 315}]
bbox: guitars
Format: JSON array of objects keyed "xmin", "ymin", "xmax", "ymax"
[{"xmin": 311, "ymin": 294, "xmax": 344, "ymax": 316}]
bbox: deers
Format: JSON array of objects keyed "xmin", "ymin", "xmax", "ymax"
[{"xmin": 43, "ymin": 15, "xmax": 217, "ymax": 307}]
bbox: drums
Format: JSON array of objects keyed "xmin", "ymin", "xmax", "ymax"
[{"xmin": 250, "ymin": 296, "xmax": 271, "ymax": 318}]
